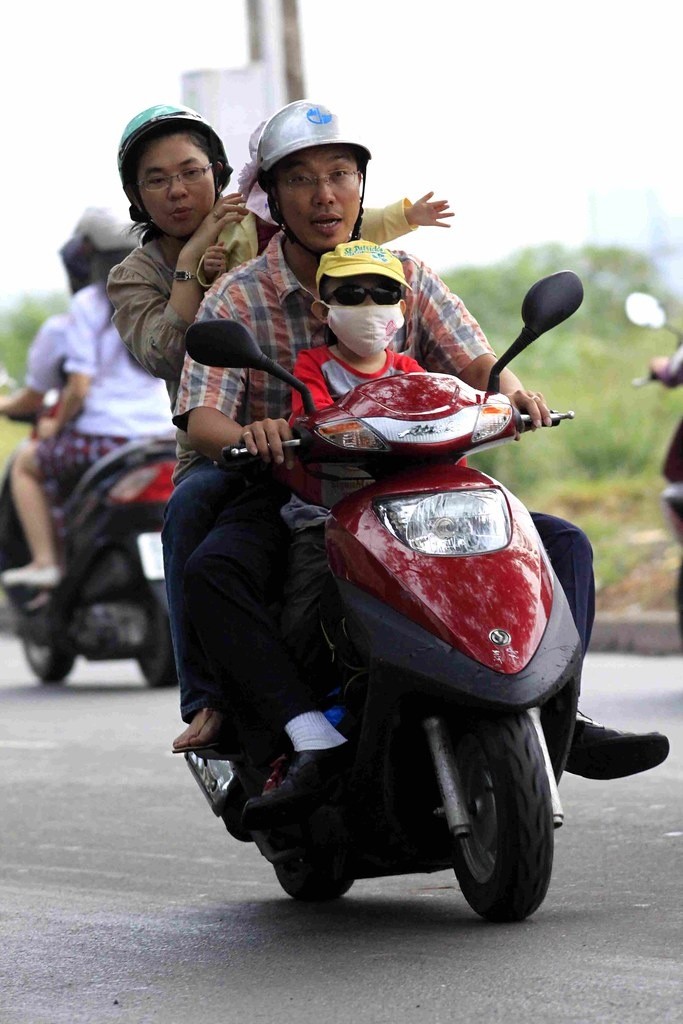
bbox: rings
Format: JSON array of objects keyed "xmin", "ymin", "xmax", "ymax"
[
  {"xmin": 532, "ymin": 396, "xmax": 538, "ymax": 400},
  {"xmin": 243, "ymin": 432, "xmax": 249, "ymax": 437},
  {"xmin": 213, "ymin": 211, "xmax": 221, "ymax": 219}
]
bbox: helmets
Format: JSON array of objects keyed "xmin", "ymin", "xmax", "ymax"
[
  {"xmin": 116, "ymin": 105, "xmax": 231, "ymax": 205},
  {"xmin": 258, "ymin": 100, "xmax": 372, "ymax": 191}
]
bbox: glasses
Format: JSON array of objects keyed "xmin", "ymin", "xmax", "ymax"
[
  {"xmin": 272, "ymin": 169, "xmax": 361, "ymax": 192},
  {"xmin": 327, "ymin": 283, "xmax": 402, "ymax": 306},
  {"xmin": 134, "ymin": 163, "xmax": 214, "ymax": 193}
]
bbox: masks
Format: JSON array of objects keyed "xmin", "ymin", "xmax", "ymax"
[{"xmin": 319, "ymin": 299, "xmax": 405, "ymax": 357}]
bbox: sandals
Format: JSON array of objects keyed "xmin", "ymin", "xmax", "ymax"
[{"xmin": 171, "ymin": 708, "xmax": 229, "ymax": 753}]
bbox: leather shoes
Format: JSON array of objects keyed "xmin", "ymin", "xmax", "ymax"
[
  {"xmin": 241, "ymin": 741, "xmax": 352, "ymax": 828},
  {"xmin": 563, "ymin": 715, "xmax": 671, "ymax": 781}
]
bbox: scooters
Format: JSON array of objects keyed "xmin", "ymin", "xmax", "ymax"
[
  {"xmin": 183, "ymin": 271, "xmax": 585, "ymax": 926},
  {"xmin": 0, "ymin": 365, "xmax": 180, "ymax": 687},
  {"xmin": 623, "ymin": 291, "xmax": 683, "ymax": 643}
]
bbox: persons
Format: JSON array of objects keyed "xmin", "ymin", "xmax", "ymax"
[
  {"xmin": 0, "ymin": 208, "xmax": 176, "ymax": 590},
  {"xmin": 277, "ymin": 239, "xmax": 468, "ymax": 661},
  {"xmin": 649, "ymin": 341, "xmax": 683, "ymax": 535},
  {"xmin": 103, "ymin": 99, "xmax": 670, "ymax": 794}
]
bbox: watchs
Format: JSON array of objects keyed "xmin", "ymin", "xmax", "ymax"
[{"xmin": 173, "ymin": 270, "xmax": 198, "ymax": 281}]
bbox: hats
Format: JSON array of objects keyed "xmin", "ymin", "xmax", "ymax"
[
  {"xmin": 58, "ymin": 206, "xmax": 141, "ymax": 255},
  {"xmin": 316, "ymin": 239, "xmax": 412, "ymax": 293}
]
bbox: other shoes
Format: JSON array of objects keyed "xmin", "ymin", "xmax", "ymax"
[
  {"xmin": 2, "ymin": 566, "xmax": 63, "ymax": 590},
  {"xmin": 261, "ymin": 753, "xmax": 293, "ymax": 794}
]
